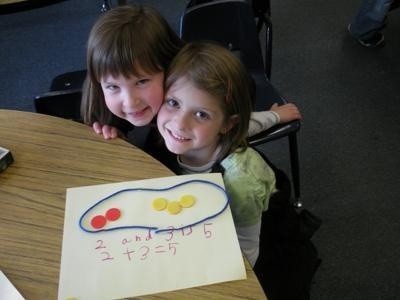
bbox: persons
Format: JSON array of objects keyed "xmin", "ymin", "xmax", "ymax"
[
  {"xmin": 347, "ymin": 0, "xmax": 400, "ymax": 48},
  {"xmin": 79, "ymin": 3, "xmax": 188, "ymax": 176},
  {"xmin": 156, "ymin": 38, "xmax": 315, "ymax": 299}
]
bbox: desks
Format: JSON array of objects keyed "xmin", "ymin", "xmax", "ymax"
[{"xmin": 0, "ymin": 108, "xmax": 269, "ymax": 300}]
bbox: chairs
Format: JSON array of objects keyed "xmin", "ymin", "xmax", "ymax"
[
  {"xmin": 177, "ymin": 0, "xmax": 304, "ymax": 209},
  {"xmin": 30, "ymin": 68, "xmax": 100, "ymax": 123}
]
